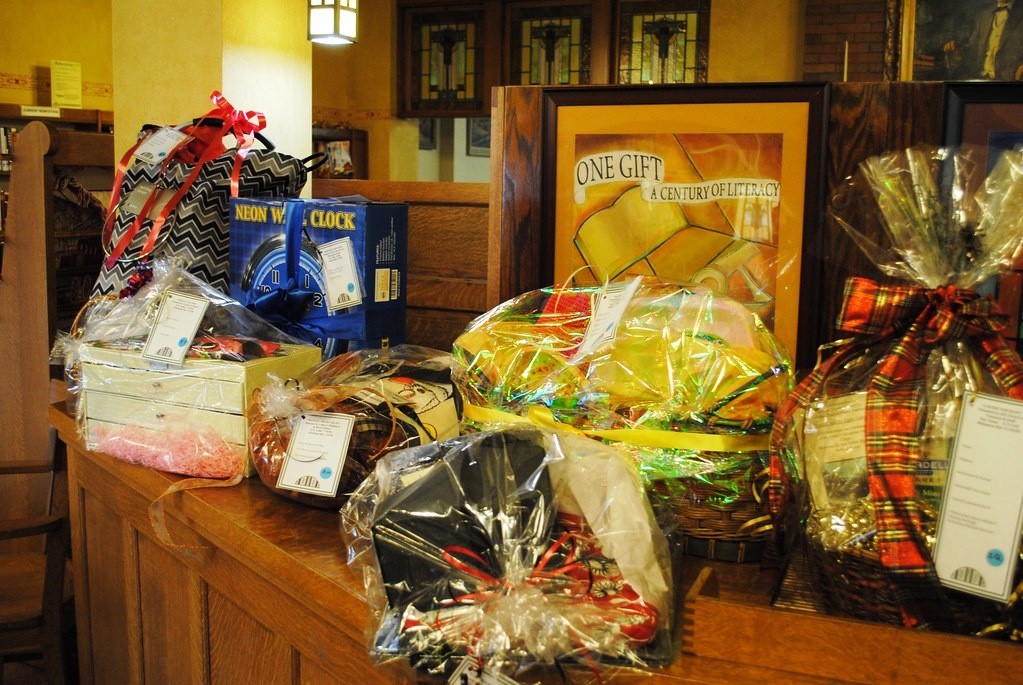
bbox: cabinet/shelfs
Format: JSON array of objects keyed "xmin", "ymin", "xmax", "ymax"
[
  {"xmin": 312, "ymin": 128, "xmax": 369, "ymax": 179},
  {"xmin": 48, "ymin": 396, "xmax": 1023, "ymax": 685}
]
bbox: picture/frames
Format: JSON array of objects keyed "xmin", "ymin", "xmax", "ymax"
[
  {"xmin": 541, "ymin": 81, "xmax": 832, "ymax": 387},
  {"xmin": 938, "ymin": 77, "xmax": 1023, "ymax": 357}
]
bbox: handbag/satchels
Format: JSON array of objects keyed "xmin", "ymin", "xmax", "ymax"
[{"xmin": 83, "ymin": 113, "xmax": 326, "ymax": 328}]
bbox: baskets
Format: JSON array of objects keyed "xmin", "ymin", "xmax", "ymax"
[
  {"xmin": 808, "ymin": 527, "xmax": 1016, "ymax": 642},
  {"xmin": 654, "ymin": 471, "xmax": 786, "ymax": 567}
]
